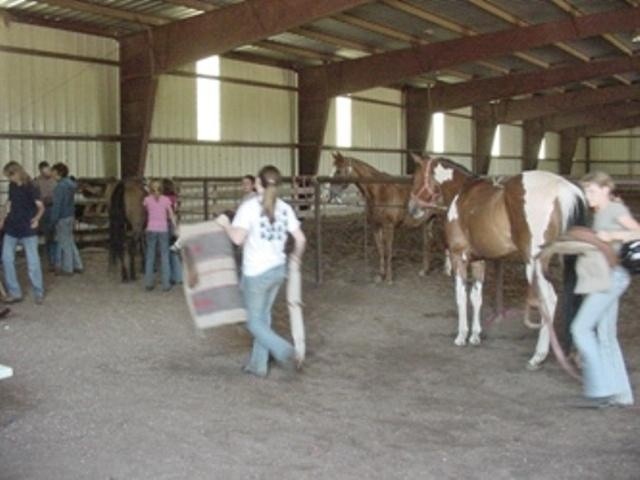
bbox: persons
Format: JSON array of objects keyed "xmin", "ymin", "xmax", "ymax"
[
  {"xmin": 0, "ymin": 159, "xmax": 46, "ymax": 304},
  {"xmin": 239, "ymin": 175, "xmax": 257, "ymax": 204},
  {"xmin": 568, "ymin": 170, "xmax": 640, "ymax": 409},
  {"xmin": 33, "ymin": 160, "xmax": 59, "ymax": 270},
  {"xmin": 162, "ymin": 179, "xmax": 184, "ymax": 285},
  {"xmin": 142, "ymin": 179, "xmax": 177, "ymax": 291},
  {"xmin": 47, "ymin": 162, "xmax": 86, "ymax": 275},
  {"xmin": 210, "ymin": 165, "xmax": 309, "ymax": 380}
]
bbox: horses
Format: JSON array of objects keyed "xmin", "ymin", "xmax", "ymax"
[
  {"xmin": 325, "ymin": 150, "xmax": 442, "ymax": 286},
  {"xmin": 400, "ymin": 148, "xmax": 593, "ymax": 371},
  {"xmin": 103, "ymin": 179, "xmax": 179, "ymax": 284}
]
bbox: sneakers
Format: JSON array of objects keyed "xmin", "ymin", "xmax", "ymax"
[
  {"xmin": 2, "ymin": 295, "xmax": 21, "ymax": 303},
  {"xmin": 567, "ymin": 395, "xmax": 615, "ymax": 408}
]
